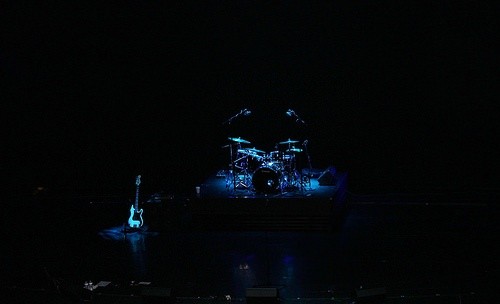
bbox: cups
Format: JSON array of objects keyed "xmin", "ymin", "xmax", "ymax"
[
  {"xmin": 85, "ymin": 284, "xmax": 88, "ymax": 288},
  {"xmin": 89, "ymin": 283, "xmax": 92, "ymax": 287},
  {"xmin": 196, "ymin": 187, "xmax": 200, "ymax": 193}
]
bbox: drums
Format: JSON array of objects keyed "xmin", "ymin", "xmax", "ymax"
[
  {"xmin": 252, "ymin": 165, "xmax": 280, "ymax": 196},
  {"xmin": 270, "ymin": 151, "xmax": 285, "ymax": 164},
  {"xmin": 283, "ymin": 155, "xmax": 296, "ymax": 170}
]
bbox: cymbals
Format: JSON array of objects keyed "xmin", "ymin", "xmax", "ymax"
[
  {"xmin": 279, "ymin": 139, "xmax": 299, "ymax": 145},
  {"xmin": 244, "ymin": 147, "xmax": 266, "ymax": 154},
  {"xmin": 228, "ymin": 136, "xmax": 251, "ymax": 145},
  {"xmin": 286, "ymin": 146, "xmax": 303, "ymax": 153}
]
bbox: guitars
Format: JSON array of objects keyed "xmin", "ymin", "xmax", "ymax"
[{"xmin": 127, "ymin": 175, "xmax": 144, "ymax": 229}]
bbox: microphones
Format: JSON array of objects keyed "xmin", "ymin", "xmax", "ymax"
[
  {"xmin": 244, "ymin": 111, "xmax": 252, "ymax": 115},
  {"xmin": 287, "ymin": 112, "xmax": 292, "ymax": 116},
  {"xmin": 303, "ymin": 140, "xmax": 309, "ymax": 146}
]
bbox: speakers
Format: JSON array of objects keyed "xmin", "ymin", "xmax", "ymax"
[
  {"xmin": 142, "ymin": 287, "xmax": 176, "ymax": 304},
  {"xmin": 352, "ymin": 287, "xmax": 387, "ymax": 304},
  {"xmin": 317, "ymin": 167, "xmax": 337, "ymax": 186},
  {"xmin": 246, "ymin": 288, "xmax": 280, "ymax": 304}
]
